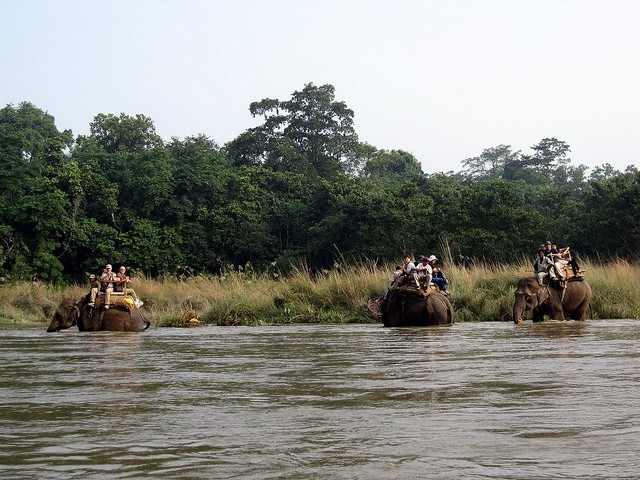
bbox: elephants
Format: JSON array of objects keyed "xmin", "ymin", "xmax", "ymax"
[
  {"xmin": 368, "ymin": 285, "xmax": 454, "ymax": 327},
  {"xmin": 46, "ymin": 294, "xmax": 151, "ymax": 332},
  {"xmin": 512, "ymin": 277, "xmax": 593, "ymax": 325}
]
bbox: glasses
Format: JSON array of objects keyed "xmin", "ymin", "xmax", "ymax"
[{"xmin": 546, "ymin": 244, "xmax": 550, "ymax": 245}]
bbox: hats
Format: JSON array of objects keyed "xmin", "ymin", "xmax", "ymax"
[
  {"xmin": 423, "ymin": 258, "xmax": 430, "ymax": 262},
  {"xmin": 106, "ymin": 264, "xmax": 112, "ymax": 269},
  {"xmin": 89, "ymin": 275, "xmax": 95, "ymax": 278},
  {"xmin": 429, "ymin": 255, "xmax": 438, "ymax": 261},
  {"xmin": 545, "ymin": 241, "xmax": 551, "ymax": 244}
]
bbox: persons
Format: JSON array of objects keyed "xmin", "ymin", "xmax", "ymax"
[
  {"xmin": 77, "ymin": 264, "xmax": 144, "ymax": 309},
  {"xmin": 375, "ymin": 255, "xmax": 451, "ymax": 296},
  {"xmin": 533, "ymin": 241, "xmax": 584, "ymax": 284}
]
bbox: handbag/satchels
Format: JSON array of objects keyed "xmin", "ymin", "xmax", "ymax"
[{"xmin": 100, "ymin": 276, "xmax": 111, "ymax": 292}]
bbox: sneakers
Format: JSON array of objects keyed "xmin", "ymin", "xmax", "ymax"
[
  {"xmin": 444, "ymin": 290, "xmax": 451, "ymax": 295},
  {"xmin": 136, "ymin": 299, "xmax": 140, "ymax": 306},
  {"xmin": 578, "ymin": 270, "xmax": 586, "ymax": 274},
  {"xmin": 104, "ymin": 305, "xmax": 109, "ymax": 309},
  {"xmin": 576, "ymin": 273, "xmax": 583, "ymax": 277},
  {"xmin": 440, "ymin": 290, "xmax": 447, "ymax": 296},
  {"xmin": 88, "ymin": 303, "xmax": 95, "ymax": 307}
]
